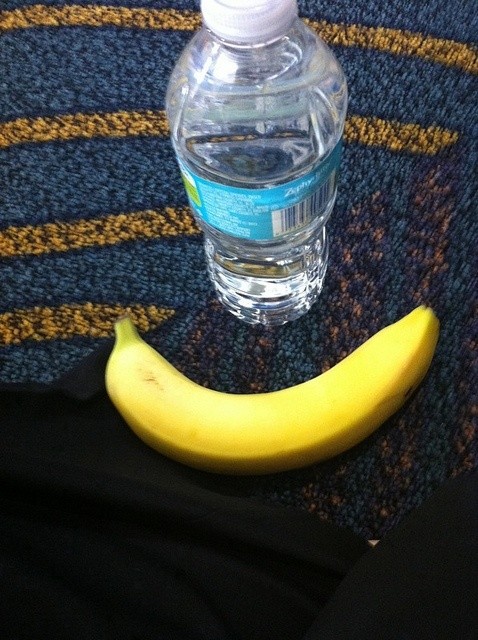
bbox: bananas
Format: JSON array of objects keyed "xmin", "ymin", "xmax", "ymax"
[{"xmin": 104, "ymin": 304, "xmax": 441, "ymax": 476}]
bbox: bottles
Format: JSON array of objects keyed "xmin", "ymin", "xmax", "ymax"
[{"xmin": 164, "ymin": 0, "xmax": 349, "ymax": 327}]
